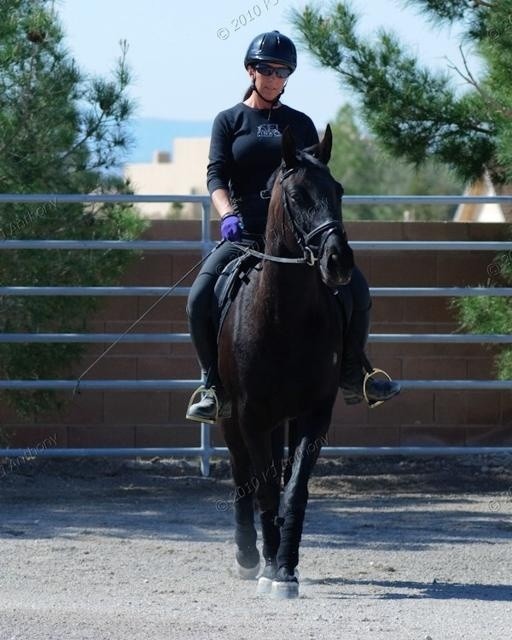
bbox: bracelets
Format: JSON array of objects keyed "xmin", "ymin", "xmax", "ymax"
[{"xmin": 221, "ymin": 211, "xmax": 237, "ymax": 221}]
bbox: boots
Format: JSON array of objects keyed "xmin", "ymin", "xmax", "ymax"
[
  {"xmin": 338, "ymin": 345, "xmax": 400, "ymax": 403},
  {"xmin": 186, "ymin": 368, "xmax": 233, "ymax": 423}
]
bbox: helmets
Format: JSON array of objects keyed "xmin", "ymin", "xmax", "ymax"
[{"xmin": 244, "ymin": 29, "xmax": 296, "ymax": 73}]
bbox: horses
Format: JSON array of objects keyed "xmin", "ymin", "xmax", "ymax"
[{"xmin": 217, "ymin": 123, "xmax": 355, "ymax": 600}]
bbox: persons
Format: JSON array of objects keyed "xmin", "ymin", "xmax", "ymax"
[{"xmin": 184, "ymin": 29, "xmax": 403, "ymax": 419}]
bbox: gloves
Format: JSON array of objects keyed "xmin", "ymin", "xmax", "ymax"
[{"xmin": 220, "ymin": 212, "xmax": 245, "ymax": 241}]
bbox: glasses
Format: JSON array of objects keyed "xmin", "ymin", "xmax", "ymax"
[{"xmin": 254, "ymin": 63, "xmax": 292, "ymax": 79}]
